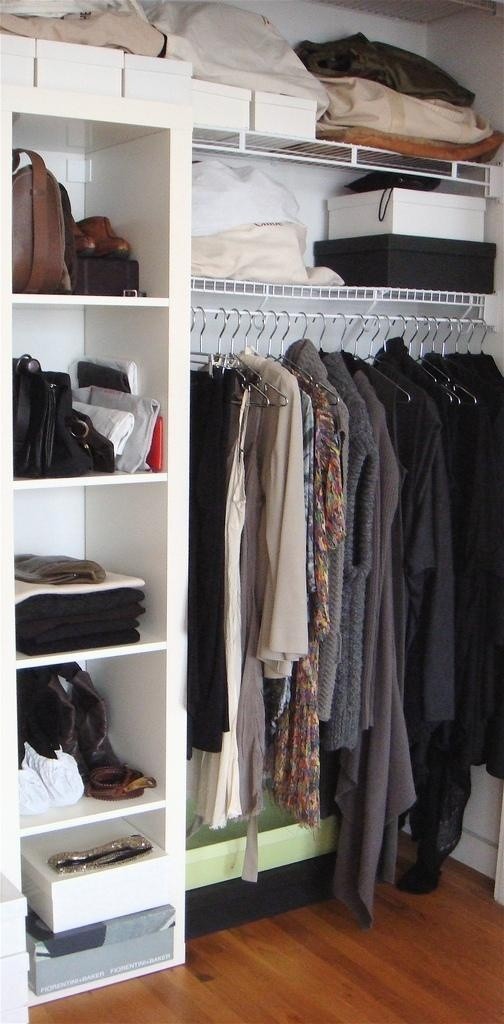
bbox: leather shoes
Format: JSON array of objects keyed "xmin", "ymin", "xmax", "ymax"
[
  {"xmin": 64, "ymin": 216, "xmax": 96, "ymax": 260},
  {"xmin": 78, "ymin": 217, "xmax": 130, "ymax": 259}
]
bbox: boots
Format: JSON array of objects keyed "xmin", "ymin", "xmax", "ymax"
[
  {"xmin": 37, "ymin": 672, "xmax": 85, "ymax": 778},
  {"xmin": 66, "ymin": 668, "xmax": 108, "ymax": 767}
]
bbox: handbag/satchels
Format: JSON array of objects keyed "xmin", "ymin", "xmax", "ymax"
[
  {"xmin": 13, "ymin": 148, "xmax": 78, "ymax": 295},
  {"xmin": 13, "ymin": 354, "xmax": 114, "ymax": 479}
]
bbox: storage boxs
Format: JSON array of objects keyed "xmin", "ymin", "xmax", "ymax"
[
  {"xmin": 251, "ymin": 92, "xmax": 321, "ymax": 141},
  {"xmin": 1, "ymin": 817, "xmax": 176, "ymax": 1024},
  {"xmin": 34, "ymin": 39, "xmax": 123, "ymax": 94},
  {"xmin": 3, "ymin": 30, "xmax": 34, "ymax": 85},
  {"xmin": 191, "ymin": 80, "xmax": 251, "ymax": 125},
  {"xmin": 326, "ymin": 188, "xmax": 487, "ymax": 241},
  {"xmin": 122, "ymin": 54, "xmax": 191, "ymax": 97},
  {"xmin": 77, "ymin": 258, "xmax": 139, "ymax": 296},
  {"xmin": 313, "ymin": 234, "xmax": 497, "ymax": 292}
]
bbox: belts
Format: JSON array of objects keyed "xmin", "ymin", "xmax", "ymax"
[{"xmin": 84, "ymin": 763, "xmax": 156, "ymax": 800}]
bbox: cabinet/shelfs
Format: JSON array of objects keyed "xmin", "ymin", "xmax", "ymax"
[{"xmin": 3, "ymin": 94, "xmax": 192, "ymax": 1009}]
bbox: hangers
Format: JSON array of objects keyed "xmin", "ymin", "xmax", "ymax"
[{"xmin": 192, "ymin": 303, "xmax": 488, "ymax": 408}]
